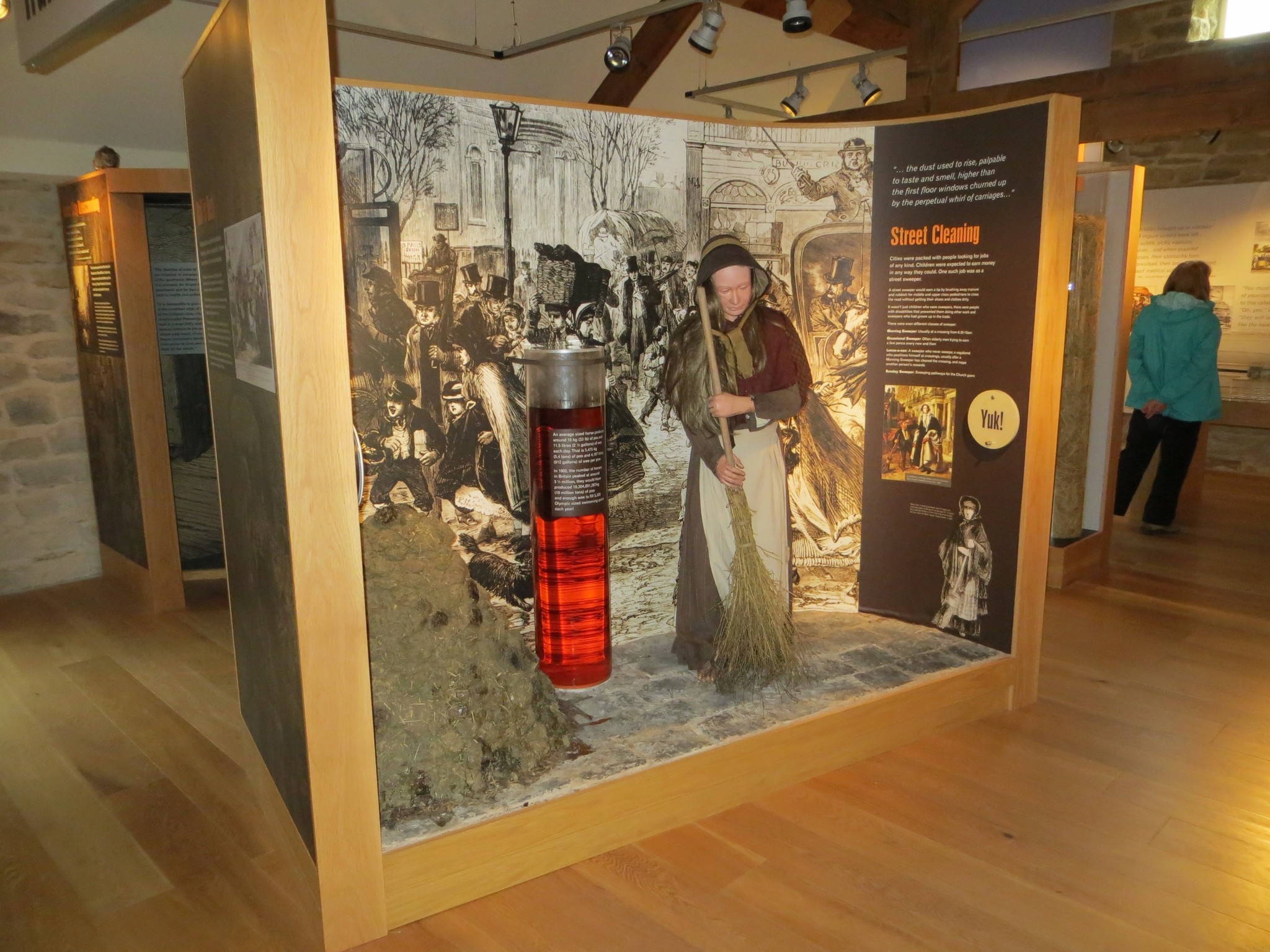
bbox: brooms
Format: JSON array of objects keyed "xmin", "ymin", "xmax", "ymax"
[{"xmin": 692, "ymin": 280, "xmax": 821, "ymax": 704}]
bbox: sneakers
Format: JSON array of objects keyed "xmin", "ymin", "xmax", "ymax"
[{"xmin": 1141, "ymin": 523, "xmax": 1179, "ymax": 536}]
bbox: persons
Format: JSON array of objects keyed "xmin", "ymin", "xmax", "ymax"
[
  {"xmin": 655, "ymin": 232, "xmax": 815, "ymax": 685},
  {"xmin": 1112, "ymin": 260, "xmax": 1223, "ymax": 537}
]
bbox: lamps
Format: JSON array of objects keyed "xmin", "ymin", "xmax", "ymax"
[
  {"xmin": 604, "ymin": 19, "xmax": 634, "ymax": 72},
  {"xmin": 1193, "ymin": 128, "xmax": 1220, "ymax": 147},
  {"xmin": 782, "ymin": 0, "xmax": 813, "ymax": 34},
  {"xmin": 851, "ymin": 62, "xmax": 882, "ymax": 106},
  {"xmin": 1, "ymin": 1, "xmax": 10, "ymax": 21},
  {"xmin": 780, "ymin": 68, "xmax": 809, "ymax": 119},
  {"xmin": 687, "ymin": 1, "xmax": 725, "ymax": 55},
  {"xmin": 1105, "ymin": 139, "xmax": 1129, "ymax": 155}
]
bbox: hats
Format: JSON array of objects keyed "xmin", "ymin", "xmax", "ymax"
[{"xmin": 693, "ymin": 235, "xmax": 773, "ymax": 310}]
